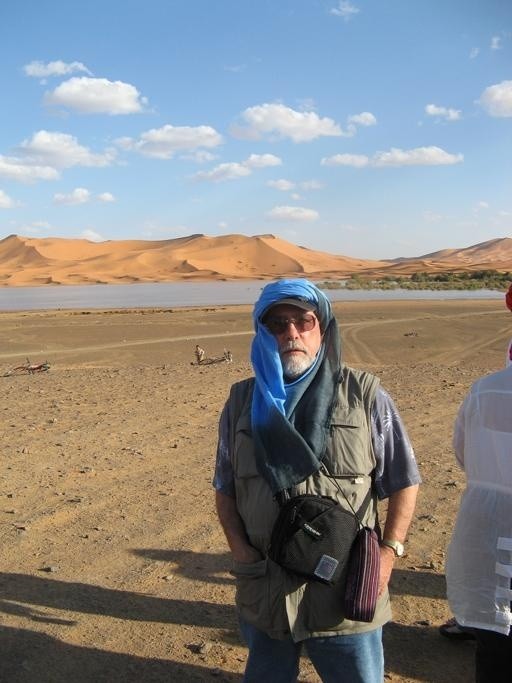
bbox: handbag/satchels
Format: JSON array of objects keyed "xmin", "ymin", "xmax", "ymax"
[{"xmin": 268, "ymin": 493, "xmax": 360, "ymax": 588}]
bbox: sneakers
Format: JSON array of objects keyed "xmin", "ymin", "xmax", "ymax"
[{"xmin": 439, "ymin": 615, "xmax": 477, "ymax": 640}]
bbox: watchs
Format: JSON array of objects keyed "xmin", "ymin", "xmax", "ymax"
[{"xmin": 381, "ymin": 536, "xmax": 406, "ymax": 557}]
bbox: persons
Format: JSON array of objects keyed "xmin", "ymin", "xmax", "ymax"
[
  {"xmin": 445, "ymin": 280, "xmax": 512, "ymax": 682},
  {"xmin": 438, "ymin": 615, "xmax": 480, "ymax": 643},
  {"xmin": 193, "ymin": 344, "xmax": 206, "ymax": 363},
  {"xmin": 211, "ymin": 274, "xmax": 422, "ymax": 682}
]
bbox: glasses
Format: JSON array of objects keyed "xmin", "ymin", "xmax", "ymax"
[{"xmin": 266, "ymin": 315, "xmax": 318, "ymax": 334}]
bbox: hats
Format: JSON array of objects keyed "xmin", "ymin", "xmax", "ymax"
[{"xmin": 259, "ymin": 298, "xmax": 318, "ymax": 321}]
